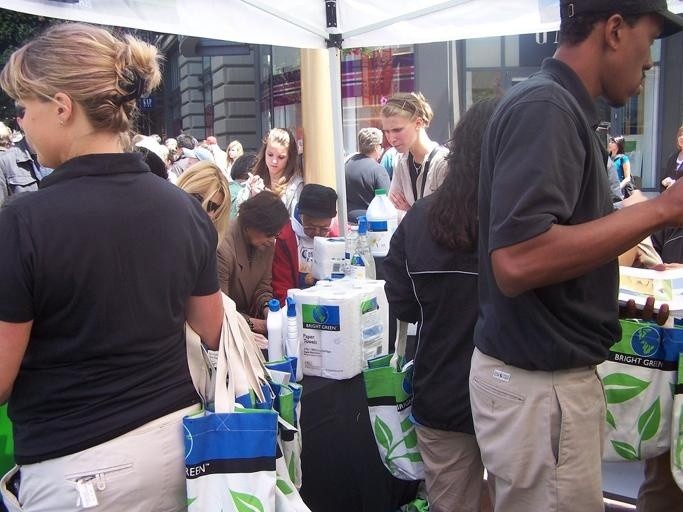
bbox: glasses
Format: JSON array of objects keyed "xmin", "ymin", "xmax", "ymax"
[
  {"xmin": 193, "ymin": 192, "xmax": 220, "ymax": 212},
  {"xmin": 265, "ymin": 231, "xmax": 280, "ymax": 237},
  {"xmin": 300, "ymin": 220, "xmax": 331, "ymax": 232}
]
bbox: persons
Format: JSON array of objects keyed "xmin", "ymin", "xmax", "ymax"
[
  {"xmin": 344, "ymin": 127, "xmax": 390, "ymax": 226},
  {"xmin": 132, "ymin": 128, "xmax": 303, "ymax": 219},
  {"xmin": 271, "ymin": 184, "xmax": 340, "ymax": 309},
  {"xmin": 2, "ymin": 24, "xmax": 224, "ymax": 511},
  {"xmin": 607, "ymin": 135, "xmax": 634, "ymax": 203},
  {"xmin": 636, "ymin": 226, "xmax": 682, "ymax": 511},
  {"xmin": 381, "ymin": 96, "xmax": 502, "ymax": 510},
  {"xmin": 380, "ymin": 92, "xmax": 451, "ymax": 227},
  {"xmin": 380, "ymin": 146, "xmax": 394, "ymax": 181},
  {"xmin": 176, "ymin": 160, "xmax": 230, "ymax": 248},
  {"xmin": 660, "ymin": 125, "xmax": 682, "ymax": 194},
  {"xmin": 470, "ymin": 1, "xmax": 683, "ymax": 511},
  {"xmin": 216, "ymin": 190, "xmax": 291, "ymax": 335},
  {"xmin": 4, "ymin": 106, "xmax": 55, "ymax": 198}
]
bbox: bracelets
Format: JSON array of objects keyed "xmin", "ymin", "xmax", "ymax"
[{"xmin": 264, "ymin": 305, "xmax": 268, "ymax": 309}]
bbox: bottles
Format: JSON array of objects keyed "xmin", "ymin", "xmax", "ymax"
[
  {"xmin": 267, "ymin": 299, "xmax": 285, "ymax": 363},
  {"xmin": 284, "ymin": 298, "xmax": 304, "ymax": 381},
  {"xmin": 366, "ymin": 189, "xmax": 397, "ymax": 253},
  {"xmin": 342, "ymin": 223, "xmax": 352, "ymax": 278},
  {"xmin": 367, "ymin": 220, "xmax": 388, "ymax": 256},
  {"xmin": 355, "ymin": 217, "xmax": 377, "ymax": 280}
]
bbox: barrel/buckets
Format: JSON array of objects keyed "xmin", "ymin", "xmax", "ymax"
[{"xmin": 365, "ymin": 189, "xmax": 398, "ymax": 257}]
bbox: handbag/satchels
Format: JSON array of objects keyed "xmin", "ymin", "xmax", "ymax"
[
  {"xmin": 364, "ymin": 355, "xmax": 424, "ymax": 482},
  {"xmin": 183, "ymin": 357, "xmax": 313, "ymax": 512},
  {"xmin": 595, "ymin": 318, "xmax": 682, "ymax": 464}
]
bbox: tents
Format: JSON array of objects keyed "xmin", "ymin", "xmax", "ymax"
[{"xmin": 0, "ymin": 1, "xmax": 683, "ymax": 265}]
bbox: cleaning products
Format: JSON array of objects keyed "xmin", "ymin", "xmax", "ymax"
[
  {"xmin": 283, "ymin": 296, "xmax": 303, "ymax": 383},
  {"xmin": 350, "ymin": 218, "xmax": 377, "ymax": 281},
  {"xmin": 266, "ymin": 300, "xmax": 284, "ymax": 363}
]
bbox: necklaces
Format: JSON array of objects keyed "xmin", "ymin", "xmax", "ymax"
[{"xmin": 413, "ymin": 156, "xmax": 424, "ymax": 174}]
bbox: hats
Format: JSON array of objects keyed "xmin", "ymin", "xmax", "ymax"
[
  {"xmin": 295, "ymin": 184, "xmax": 337, "ymax": 219},
  {"xmin": 182, "ymin": 147, "xmax": 215, "ymax": 163},
  {"xmin": 559, "ymin": 0, "xmax": 682, "ymax": 38}
]
